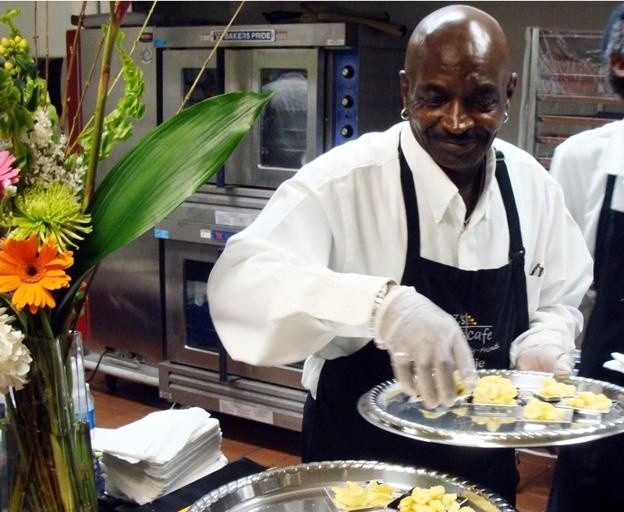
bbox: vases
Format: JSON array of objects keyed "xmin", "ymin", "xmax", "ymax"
[{"xmin": 1, "ymin": 329, "xmax": 96, "ymax": 510}]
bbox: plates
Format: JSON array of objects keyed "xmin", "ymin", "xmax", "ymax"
[
  {"xmin": 177, "ymin": 459, "xmax": 515, "ymax": 512},
  {"xmin": 357, "ymin": 364, "xmax": 623, "ymax": 448}
]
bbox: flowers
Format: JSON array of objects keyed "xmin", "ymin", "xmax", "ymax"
[{"xmin": 1, "ymin": 2, "xmax": 279, "ymax": 512}]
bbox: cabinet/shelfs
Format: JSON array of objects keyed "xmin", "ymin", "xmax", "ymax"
[{"xmin": 516, "ymin": 26, "xmax": 624, "ymax": 171}]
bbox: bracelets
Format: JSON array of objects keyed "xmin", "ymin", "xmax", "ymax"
[{"xmin": 371, "ymin": 284, "xmax": 388, "ymax": 328}]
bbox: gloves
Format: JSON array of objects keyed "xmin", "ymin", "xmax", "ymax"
[
  {"xmin": 509, "ymin": 304, "xmax": 584, "ymax": 379},
  {"xmin": 602, "ymin": 352, "xmax": 624, "ymax": 373},
  {"xmin": 374, "ymin": 285, "xmax": 477, "ymax": 410}
]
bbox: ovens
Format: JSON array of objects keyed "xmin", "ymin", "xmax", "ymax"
[{"xmin": 72, "ymin": 19, "xmax": 404, "ymax": 438}]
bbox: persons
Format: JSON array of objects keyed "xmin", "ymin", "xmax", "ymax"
[
  {"xmin": 546, "ymin": 1, "xmax": 624, "ymax": 512},
  {"xmin": 206, "ymin": 4, "xmax": 595, "ymax": 512}
]
